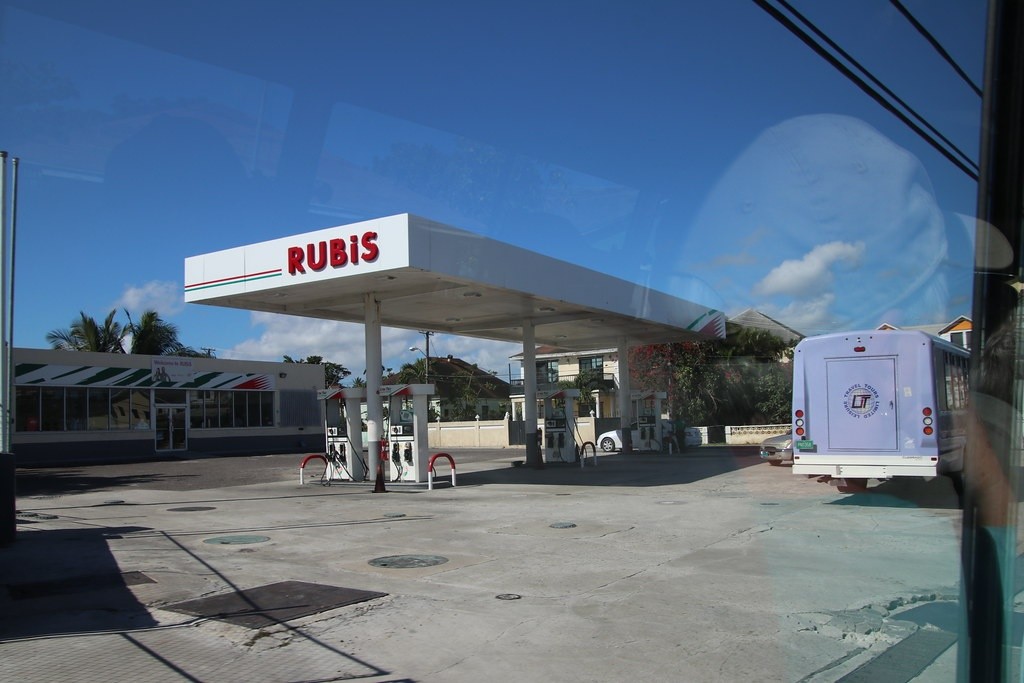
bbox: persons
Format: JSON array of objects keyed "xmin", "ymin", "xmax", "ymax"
[{"xmin": 668, "ymin": 413, "xmax": 687, "ymax": 455}]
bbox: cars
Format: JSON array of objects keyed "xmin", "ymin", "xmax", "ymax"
[
  {"xmin": 759, "ymin": 429, "xmax": 793, "ymax": 465},
  {"xmin": 597, "ymin": 419, "xmax": 702, "ymax": 452}
]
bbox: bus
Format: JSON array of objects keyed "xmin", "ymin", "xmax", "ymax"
[{"xmin": 792, "ymin": 330, "xmax": 971, "ymax": 493}]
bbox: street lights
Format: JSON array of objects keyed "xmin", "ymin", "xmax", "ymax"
[{"xmin": 410, "ymin": 347, "xmax": 429, "ymax": 384}]
